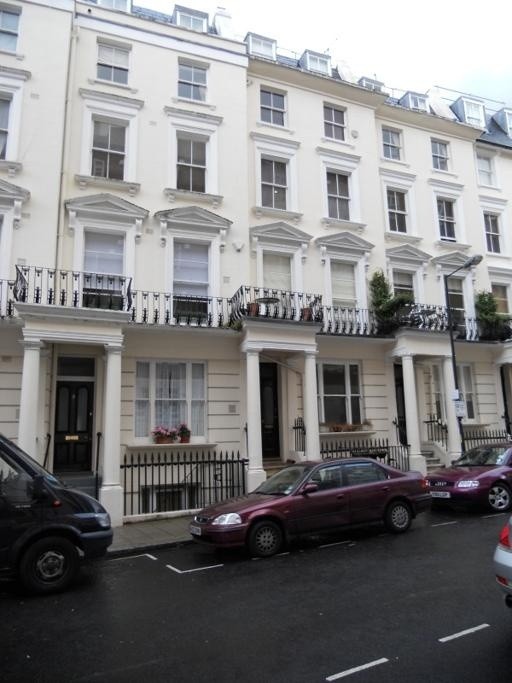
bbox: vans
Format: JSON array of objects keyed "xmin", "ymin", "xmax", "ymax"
[{"xmin": 0, "ymin": 435, "xmax": 113, "ymax": 601}]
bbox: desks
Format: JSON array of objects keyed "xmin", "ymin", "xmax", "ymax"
[{"xmin": 254, "ymin": 297, "xmax": 280, "ymax": 317}]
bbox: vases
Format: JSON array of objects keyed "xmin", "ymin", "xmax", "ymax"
[
  {"xmin": 181, "ymin": 436, "xmax": 189, "ymax": 443},
  {"xmin": 156, "ymin": 437, "xmax": 173, "ymax": 444}
]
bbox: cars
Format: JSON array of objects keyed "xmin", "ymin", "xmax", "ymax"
[
  {"xmin": 189, "ymin": 457, "xmax": 435, "ymax": 558},
  {"xmin": 493, "ymin": 511, "xmax": 512, "ymax": 606},
  {"xmin": 424, "ymin": 440, "xmax": 512, "ymax": 521}
]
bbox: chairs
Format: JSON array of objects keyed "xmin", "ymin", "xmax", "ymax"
[
  {"xmin": 246, "ymin": 303, "xmax": 260, "ymax": 316},
  {"xmin": 299, "ymin": 308, "xmax": 313, "ymax": 320}
]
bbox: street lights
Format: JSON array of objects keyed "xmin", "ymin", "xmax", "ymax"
[{"xmin": 441, "ymin": 252, "xmax": 483, "ymax": 455}]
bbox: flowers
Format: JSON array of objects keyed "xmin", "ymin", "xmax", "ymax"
[
  {"xmin": 177, "ymin": 422, "xmax": 192, "ymax": 438},
  {"xmin": 148, "ymin": 424, "xmax": 178, "ymax": 441}
]
